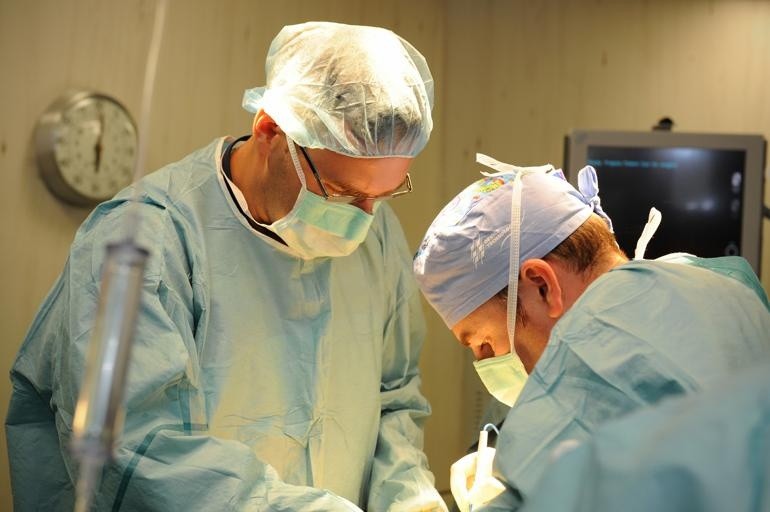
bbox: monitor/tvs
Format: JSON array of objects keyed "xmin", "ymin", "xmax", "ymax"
[{"xmin": 563, "ymin": 130, "xmax": 768, "ymax": 282}]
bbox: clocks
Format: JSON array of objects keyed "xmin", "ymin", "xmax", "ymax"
[{"xmin": 33, "ymin": 92, "xmax": 140, "ymax": 208}]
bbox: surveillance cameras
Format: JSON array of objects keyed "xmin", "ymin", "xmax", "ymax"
[{"xmin": 653, "ymin": 118, "xmax": 673, "ymax": 131}]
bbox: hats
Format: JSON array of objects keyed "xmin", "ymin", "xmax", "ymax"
[
  {"xmin": 241, "ymin": 21, "xmax": 434, "ymax": 159},
  {"xmin": 413, "ymin": 173, "xmax": 615, "ymax": 330}
]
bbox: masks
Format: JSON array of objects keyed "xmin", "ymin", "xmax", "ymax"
[
  {"xmin": 220, "ymin": 133, "xmax": 381, "ymax": 259},
  {"xmin": 472, "ymin": 153, "xmax": 555, "ymax": 408}
]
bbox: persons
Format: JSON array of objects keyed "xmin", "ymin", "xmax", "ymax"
[
  {"xmin": 6, "ymin": 22, "xmax": 449, "ymax": 510},
  {"xmin": 412, "ymin": 171, "xmax": 770, "ymax": 512}
]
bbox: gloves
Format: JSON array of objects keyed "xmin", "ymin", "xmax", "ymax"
[{"xmin": 450, "ymin": 446, "xmax": 506, "ymax": 512}]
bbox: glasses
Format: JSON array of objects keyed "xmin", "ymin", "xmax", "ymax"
[{"xmin": 300, "ymin": 145, "xmax": 414, "ymax": 206}]
